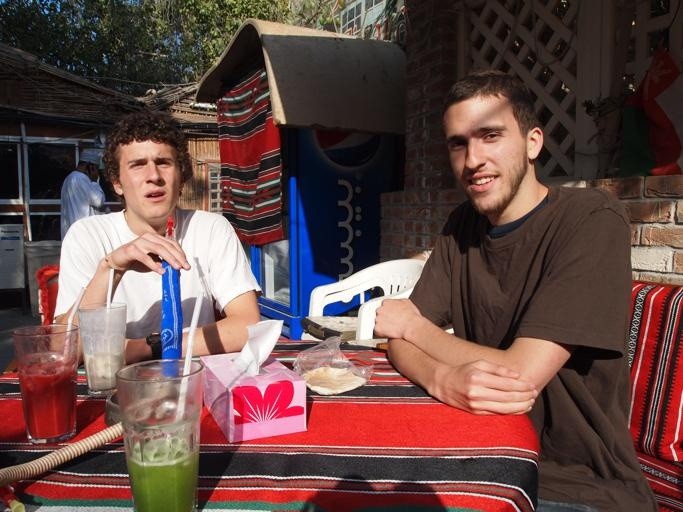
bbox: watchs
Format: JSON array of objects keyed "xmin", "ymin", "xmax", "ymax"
[{"xmin": 145, "ymin": 331, "xmax": 163, "ymax": 357}]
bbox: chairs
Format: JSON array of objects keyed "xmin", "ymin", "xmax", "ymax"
[
  {"xmin": 619, "ymin": 278, "xmax": 682, "ymax": 511},
  {"xmin": 300, "ymin": 257, "xmax": 430, "ymax": 348}
]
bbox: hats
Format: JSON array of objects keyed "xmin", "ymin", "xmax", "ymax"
[{"xmin": 79, "ymin": 150, "xmax": 100, "ymax": 166}]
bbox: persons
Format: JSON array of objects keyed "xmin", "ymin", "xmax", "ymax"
[
  {"xmin": 59, "ymin": 150, "xmax": 106, "ymax": 243},
  {"xmin": 373, "ymin": 71, "xmax": 659, "ymax": 511},
  {"xmin": 52, "ymin": 110, "xmax": 262, "ymax": 364}
]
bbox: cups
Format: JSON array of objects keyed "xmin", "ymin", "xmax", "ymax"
[
  {"xmin": 12, "ymin": 323, "xmax": 80, "ymax": 445},
  {"xmin": 114, "ymin": 359, "xmax": 204, "ymax": 512},
  {"xmin": 75, "ymin": 301, "xmax": 129, "ymax": 399}
]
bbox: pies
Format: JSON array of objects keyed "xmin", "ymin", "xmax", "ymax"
[{"xmin": 302, "ymin": 366, "xmax": 369, "ymax": 396}]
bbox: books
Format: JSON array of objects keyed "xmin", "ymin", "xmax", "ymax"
[{"xmin": 301, "ymin": 314, "xmax": 357, "ymax": 342}]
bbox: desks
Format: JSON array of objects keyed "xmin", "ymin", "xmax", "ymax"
[{"xmin": 0, "ymin": 341, "xmax": 547, "ymax": 510}]
